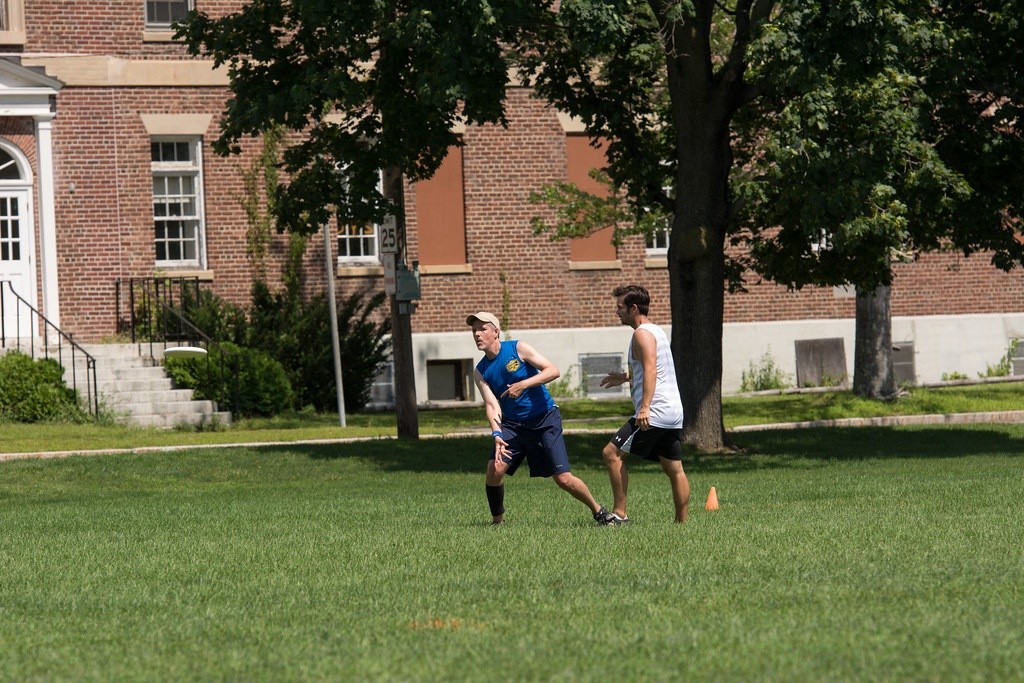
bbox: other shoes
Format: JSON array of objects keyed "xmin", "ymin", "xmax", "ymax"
[{"xmin": 594, "ymin": 507, "xmax": 609, "ymax": 522}]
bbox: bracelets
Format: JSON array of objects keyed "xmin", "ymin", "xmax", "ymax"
[{"xmin": 492, "ymin": 431, "xmax": 503, "ymax": 437}]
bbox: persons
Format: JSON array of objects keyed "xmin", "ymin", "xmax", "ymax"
[
  {"xmin": 600, "ymin": 286, "xmax": 690, "ymax": 524},
  {"xmin": 466, "ymin": 311, "xmax": 614, "ymax": 525}
]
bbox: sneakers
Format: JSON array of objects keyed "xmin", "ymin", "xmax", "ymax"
[{"xmin": 596, "ymin": 513, "xmax": 630, "ymax": 526}]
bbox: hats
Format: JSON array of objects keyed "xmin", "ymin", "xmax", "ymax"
[{"xmin": 466, "ymin": 312, "xmax": 499, "ymax": 330}]
